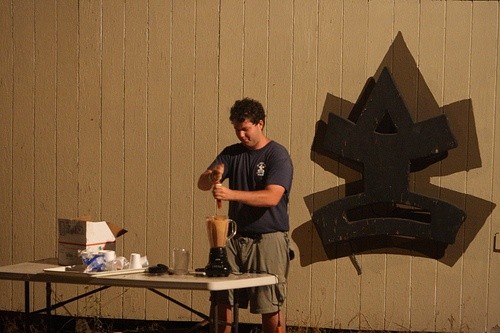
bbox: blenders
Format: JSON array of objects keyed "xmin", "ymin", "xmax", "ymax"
[{"xmin": 204, "ymin": 214, "xmax": 237, "ymax": 277}]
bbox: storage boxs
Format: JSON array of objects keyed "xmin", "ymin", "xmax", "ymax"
[{"xmin": 57, "ymin": 216, "xmax": 128, "ymax": 265}]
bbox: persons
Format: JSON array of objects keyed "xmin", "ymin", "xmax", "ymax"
[{"xmin": 196, "ymin": 97, "xmax": 294, "ymax": 333}]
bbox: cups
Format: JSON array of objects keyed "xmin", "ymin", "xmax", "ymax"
[
  {"xmin": 173, "ymin": 249, "xmax": 190, "ymax": 274},
  {"xmin": 99, "ymin": 250, "xmax": 114, "ymax": 261},
  {"xmin": 129, "ymin": 253, "xmax": 142, "ymax": 269}
]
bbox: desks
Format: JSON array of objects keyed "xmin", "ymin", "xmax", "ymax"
[{"xmin": 0, "ymin": 257, "xmax": 278, "ymax": 333}]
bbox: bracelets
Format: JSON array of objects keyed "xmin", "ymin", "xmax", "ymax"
[{"xmin": 208, "ymin": 172, "xmax": 214, "ymax": 184}]
